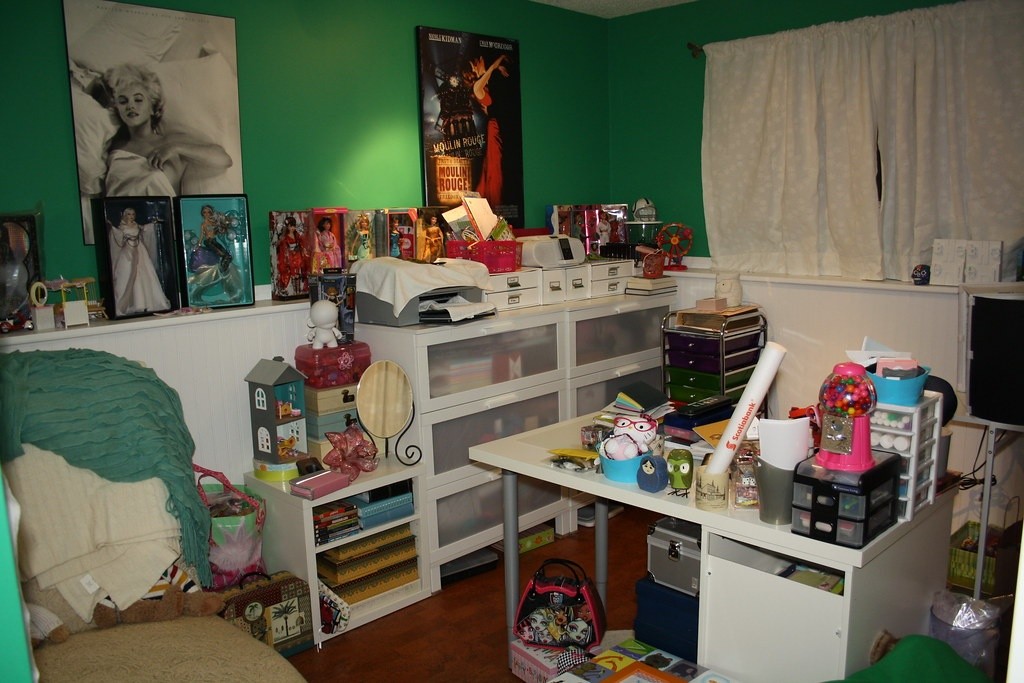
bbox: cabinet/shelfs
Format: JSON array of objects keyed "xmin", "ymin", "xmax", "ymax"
[
  {"xmin": 243, "ymin": 453, "xmax": 432, "ymax": 645},
  {"xmin": 871, "ymin": 389, "xmax": 944, "ymax": 521},
  {"xmin": 352, "ymin": 292, "xmax": 677, "ymax": 595},
  {"xmin": 660, "ymin": 306, "xmax": 767, "ymax": 418}
]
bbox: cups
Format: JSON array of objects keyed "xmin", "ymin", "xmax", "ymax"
[{"xmin": 694, "ymin": 465, "xmax": 730, "ymax": 513}]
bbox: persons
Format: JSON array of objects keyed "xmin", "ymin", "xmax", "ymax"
[
  {"xmin": 279, "ymin": 217, "xmax": 304, "ymax": 285},
  {"xmin": 351, "ymin": 218, "xmax": 371, "ymax": 260},
  {"xmin": 195, "ymin": 205, "xmax": 226, "ymax": 250},
  {"xmin": 317, "ymin": 217, "xmax": 342, "ymax": 268},
  {"xmin": 307, "ymin": 300, "xmax": 342, "ymax": 349},
  {"xmin": 389, "ymin": 216, "xmax": 404, "ymax": 259},
  {"xmin": 109, "ymin": 208, "xmax": 171, "ymax": 315},
  {"xmin": 104, "ymin": 64, "xmax": 232, "ymax": 197},
  {"xmin": 471, "ymin": 54, "xmax": 509, "ymax": 211},
  {"xmin": 425, "ymin": 214, "xmax": 443, "ymax": 263}
]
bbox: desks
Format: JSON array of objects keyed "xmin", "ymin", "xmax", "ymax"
[{"xmin": 468, "ymin": 409, "xmax": 961, "ymax": 683}]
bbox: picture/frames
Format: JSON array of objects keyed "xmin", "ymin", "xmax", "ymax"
[
  {"xmin": 173, "ymin": 194, "xmax": 255, "ymax": 308},
  {"xmin": 91, "ymin": 197, "xmax": 180, "ymax": 319}
]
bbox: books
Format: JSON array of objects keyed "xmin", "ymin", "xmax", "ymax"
[
  {"xmin": 614, "ymin": 381, "xmax": 668, "ymax": 413},
  {"xmin": 625, "ymin": 277, "xmax": 678, "ymax": 296}
]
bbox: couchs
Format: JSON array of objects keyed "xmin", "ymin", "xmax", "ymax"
[{"xmin": 1, "ymin": 347, "xmax": 307, "ymax": 683}]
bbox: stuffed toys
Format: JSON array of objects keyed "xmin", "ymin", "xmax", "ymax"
[
  {"xmin": 603, "ymin": 413, "xmax": 658, "ymax": 460},
  {"xmin": 93, "ymin": 564, "xmax": 225, "ymax": 628},
  {"xmin": 26, "ymin": 603, "xmax": 70, "ymax": 648}
]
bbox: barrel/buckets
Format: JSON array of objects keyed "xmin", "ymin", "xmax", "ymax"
[
  {"xmin": 624, "ymin": 221, "xmax": 663, "ymax": 246},
  {"xmin": 752, "ymin": 457, "xmax": 794, "ymax": 526}
]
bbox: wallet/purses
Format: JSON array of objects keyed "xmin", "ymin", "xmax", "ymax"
[{"xmin": 290, "ymin": 469, "xmax": 350, "ymax": 500}]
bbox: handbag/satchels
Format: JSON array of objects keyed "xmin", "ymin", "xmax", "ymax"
[
  {"xmin": 220, "ymin": 571, "xmax": 313, "ymax": 657},
  {"xmin": 192, "ymin": 464, "xmax": 265, "ymax": 591},
  {"xmin": 513, "ymin": 558, "xmax": 606, "ymax": 652}
]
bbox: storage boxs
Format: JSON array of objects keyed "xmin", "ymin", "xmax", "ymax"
[
  {"xmin": 447, "ymin": 239, "xmax": 517, "ymax": 274},
  {"xmin": 487, "ymin": 259, "xmax": 635, "ymax": 311},
  {"xmin": 547, "ymin": 638, "xmax": 734, "ymax": 683},
  {"xmin": 510, "ymin": 639, "xmax": 603, "ymax": 683},
  {"xmin": 646, "ymin": 516, "xmax": 701, "ymax": 598},
  {"xmin": 947, "ymin": 518, "xmax": 1005, "ymax": 595},
  {"xmin": 791, "ymin": 449, "xmax": 899, "ymax": 550},
  {"xmin": 295, "ymin": 339, "xmax": 384, "ymax": 467}
]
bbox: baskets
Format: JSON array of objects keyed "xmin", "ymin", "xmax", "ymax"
[{"xmin": 446, "ymin": 240, "xmax": 523, "ymax": 273}]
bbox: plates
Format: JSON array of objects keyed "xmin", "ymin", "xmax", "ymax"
[{"xmin": 355, "ymin": 359, "xmax": 413, "ymax": 439}]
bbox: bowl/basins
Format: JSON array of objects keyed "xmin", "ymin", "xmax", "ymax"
[{"xmin": 595, "ymin": 442, "xmax": 653, "ymax": 484}]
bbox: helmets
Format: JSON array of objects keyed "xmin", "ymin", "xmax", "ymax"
[{"xmin": 632, "ymin": 198, "xmax": 656, "ymax": 221}]
